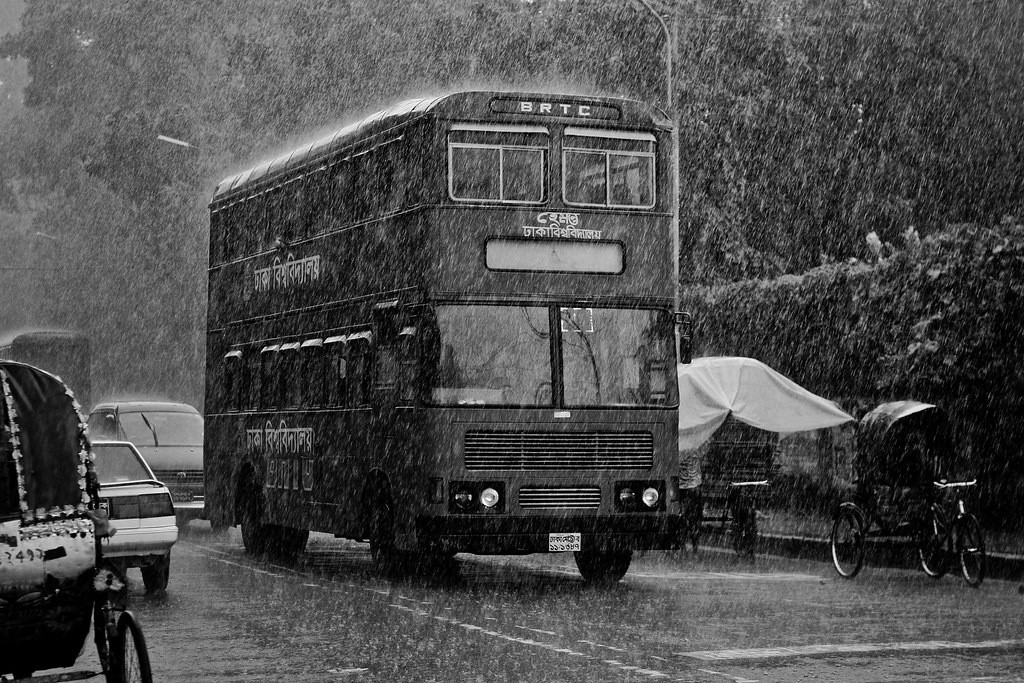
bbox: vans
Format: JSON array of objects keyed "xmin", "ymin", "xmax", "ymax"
[{"xmin": 90, "ymin": 401, "xmax": 205, "ymax": 526}]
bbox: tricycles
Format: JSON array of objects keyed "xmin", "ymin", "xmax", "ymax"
[
  {"xmin": 0, "ymin": 360, "xmax": 152, "ymax": 683},
  {"xmin": 675, "ymin": 356, "xmax": 852, "ymax": 553},
  {"xmin": 830, "ymin": 401, "xmax": 986, "ymax": 589}
]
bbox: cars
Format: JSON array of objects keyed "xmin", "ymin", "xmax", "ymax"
[{"xmin": 88, "ymin": 438, "xmax": 178, "ymax": 594}]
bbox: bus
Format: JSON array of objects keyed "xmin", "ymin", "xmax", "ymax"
[
  {"xmin": 0, "ymin": 329, "xmax": 91, "ymax": 409},
  {"xmin": 201, "ymin": 93, "xmax": 681, "ymax": 588}
]
